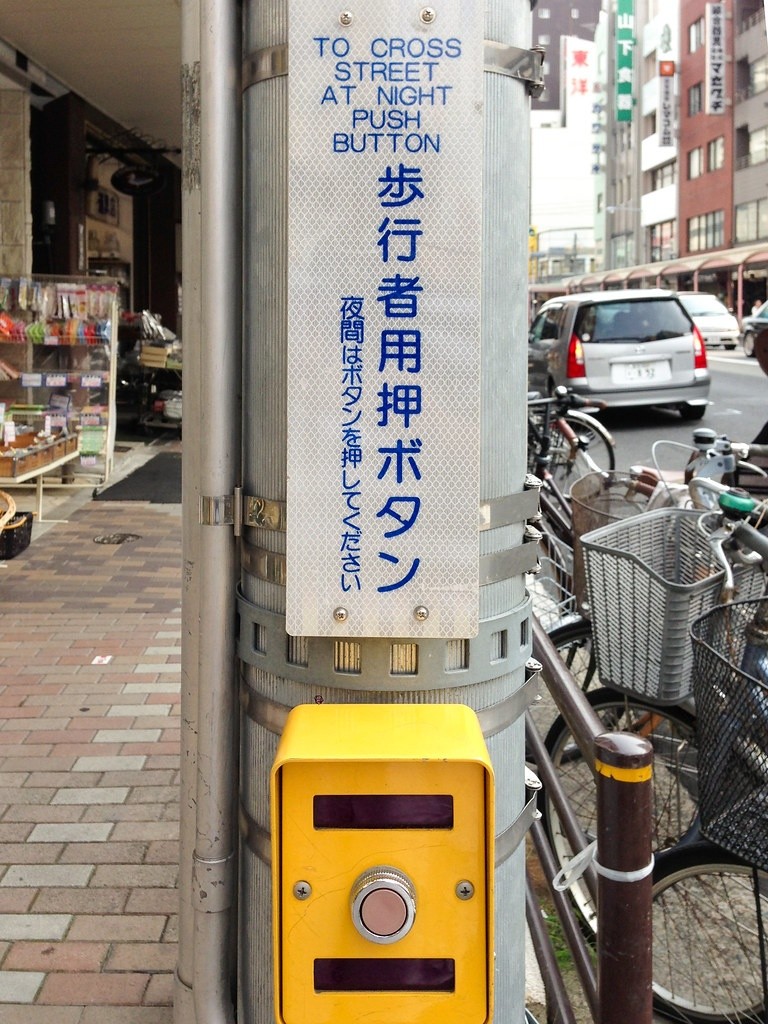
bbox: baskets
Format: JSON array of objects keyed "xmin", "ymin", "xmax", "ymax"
[
  {"xmin": 686, "ymin": 591, "xmax": 768, "ymax": 868},
  {"xmin": 568, "ymin": 468, "xmax": 659, "ymax": 618},
  {"xmin": 576, "ymin": 506, "xmax": 766, "ymax": 711},
  {"xmin": 0, "ymin": 510, "xmax": 34, "ymax": 560}
]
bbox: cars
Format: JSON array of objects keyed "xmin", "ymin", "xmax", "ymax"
[
  {"xmin": 740, "ymin": 300, "xmax": 768, "ymax": 358},
  {"xmin": 677, "ymin": 291, "xmax": 742, "ymax": 351},
  {"xmin": 529, "ymin": 289, "xmax": 711, "ymax": 423}
]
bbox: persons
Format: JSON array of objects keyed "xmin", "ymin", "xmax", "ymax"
[{"xmin": 751, "ymin": 299, "xmax": 764, "ymax": 316}]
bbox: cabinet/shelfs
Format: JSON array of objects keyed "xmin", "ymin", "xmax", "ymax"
[{"xmin": 0, "ymin": 271, "xmax": 122, "ymax": 526}]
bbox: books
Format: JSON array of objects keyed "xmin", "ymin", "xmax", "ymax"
[{"xmin": 139, "ymin": 344, "xmax": 169, "ymax": 368}]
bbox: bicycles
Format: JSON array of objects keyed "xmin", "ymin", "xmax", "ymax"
[{"xmin": 531, "ymin": 386, "xmax": 768, "ymax": 1023}]
